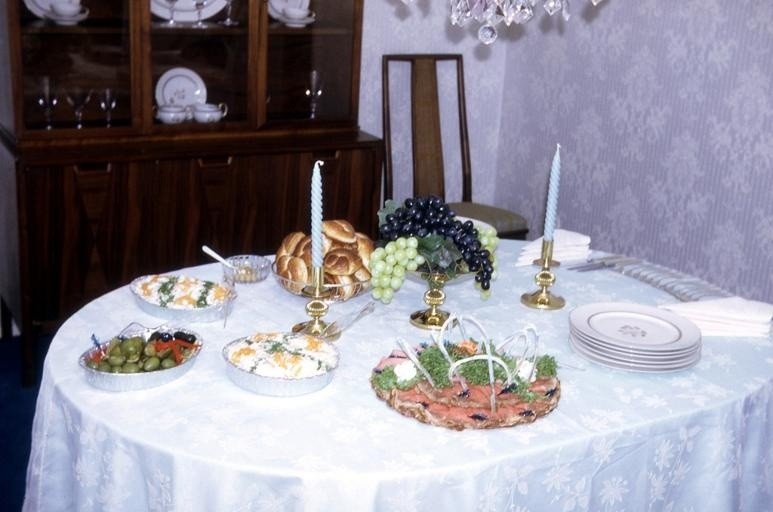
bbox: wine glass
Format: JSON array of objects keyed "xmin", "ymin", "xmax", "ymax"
[
  {"xmin": 34, "ymin": 76, "xmax": 120, "ymax": 130},
  {"xmin": 303, "ymin": 72, "xmax": 322, "ymax": 122},
  {"xmin": 159, "ymin": 1, "xmax": 240, "ymax": 29}
]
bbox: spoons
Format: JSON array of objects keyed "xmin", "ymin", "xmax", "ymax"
[{"xmin": 199, "ymin": 244, "xmax": 252, "ymax": 280}]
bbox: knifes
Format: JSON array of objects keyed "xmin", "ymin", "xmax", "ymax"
[{"xmin": 565, "ymin": 253, "xmax": 645, "ymax": 277}]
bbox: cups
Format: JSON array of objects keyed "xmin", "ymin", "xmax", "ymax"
[
  {"xmin": 284, "ymin": 8, "xmax": 314, "ymax": 21},
  {"xmin": 50, "ymin": 5, "xmax": 87, "ymax": 18}
]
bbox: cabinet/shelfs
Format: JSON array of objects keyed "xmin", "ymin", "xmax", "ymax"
[{"xmin": 0, "ymin": 1, "xmax": 383, "ymax": 388}]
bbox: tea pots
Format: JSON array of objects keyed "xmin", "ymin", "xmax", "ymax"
[
  {"xmin": 194, "ymin": 103, "xmax": 228, "ymax": 122},
  {"xmin": 153, "ymin": 100, "xmax": 190, "ymax": 125}
]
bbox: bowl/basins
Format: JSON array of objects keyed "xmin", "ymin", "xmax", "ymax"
[{"xmin": 222, "ymin": 255, "xmax": 273, "ymax": 282}]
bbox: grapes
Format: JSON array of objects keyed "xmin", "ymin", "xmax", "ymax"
[
  {"xmin": 466, "ymin": 223, "xmax": 499, "ymax": 298},
  {"xmin": 367, "ymin": 236, "xmax": 424, "ymax": 305},
  {"xmin": 381, "ymin": 195, "xmax": 494, "ymax": 292}
]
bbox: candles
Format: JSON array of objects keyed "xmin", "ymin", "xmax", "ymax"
[
  {"xmin": 309, "ymin": 161, "xmax": 325, "ymax": 266},
  {"xmin": 542, "ymin": 144, "xmax": 563, "ymax": 239}
]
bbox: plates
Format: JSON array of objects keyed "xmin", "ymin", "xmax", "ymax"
[
  {"xmin": 568, "ymin": 303, "xmax": 700, "ymax": 369},
  {"xmin": 222, "ymin": 334, "xmax": 338, "ymax": 397},
  {"xmin": 155, "ymin": 68, "xmax": 207, "ymax": 108},
  {"xmin": 45, "ymin": 13, "xmax": 90, "ymax": 25},
  {"xmin": 77, "ymin": 326, "xmax": 201, "ymax": 391},
  {"xmin": 279, "ymin": 16, "xmax": 316, "ymax": 30},
  {"xmin": 150, "ymin": 1, "xmax": 225, "ymax": 21},
  {"xmin": 130, "ymin": 272, "xmax": 236, "ymax": 325},
  {"xmin": 269, "ymin": 262, "xmax": 375, "ymax": 300},
  {"xmin": 267, "ymin": 1, "xmax": 310, "ymax": 20},
  {"xmin": 23, "ymin": 0, "xmax": 81, "ymax": 18}
]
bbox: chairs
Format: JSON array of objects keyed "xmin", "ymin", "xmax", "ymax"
[{"xmin": 380, "ymin": 53, "xmax": 529, "ymax": 243}]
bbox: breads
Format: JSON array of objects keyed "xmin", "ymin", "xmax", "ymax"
[{"xmin": 277, "ymin": 217, "xmax": 375, "ymax": 300}]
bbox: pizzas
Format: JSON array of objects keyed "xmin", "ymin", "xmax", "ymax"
[{"xmin": 372, "ymin": 341, "xmax": 560, "ymax": 429}]
